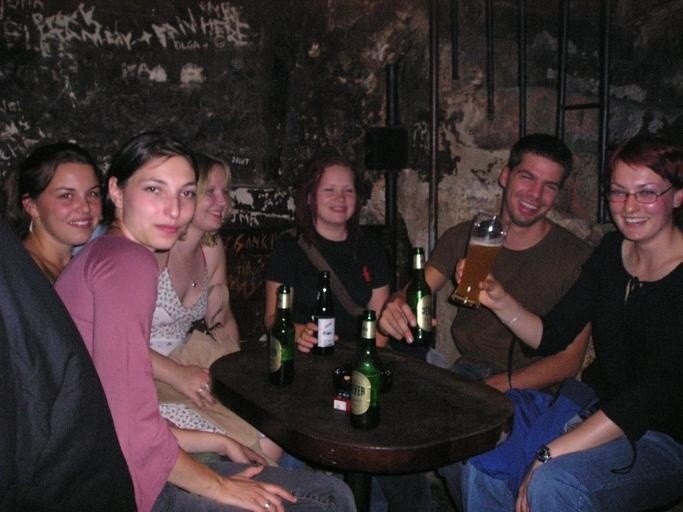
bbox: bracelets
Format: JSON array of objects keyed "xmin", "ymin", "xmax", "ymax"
[{"xmin": 505, "ymin": 305, "xmax": 524, "ymax": 328}]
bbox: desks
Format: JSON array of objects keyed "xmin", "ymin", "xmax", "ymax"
[{"xmin": 208, "ymin": 333, "xmax": 514, "ymax": 512}]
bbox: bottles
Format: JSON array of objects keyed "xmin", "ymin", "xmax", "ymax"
[
  {"xmin": 405, "ymin": 247, "xmax": 434, "ymax": 346},
  {"xmin": 312, "ymin": 270, "xmax": 335, "ymax": 350},
  {"xmin": 349, "ymin": 309, "xmax": 380, "ymax": 428},
  {"xmin": 268, "ymin": 284, "xmax": 294, "ymax": 387}
]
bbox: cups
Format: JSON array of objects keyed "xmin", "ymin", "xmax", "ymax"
[{"xmin": 450, "ymin": 213, "xmax": 511, "ymax": 309}]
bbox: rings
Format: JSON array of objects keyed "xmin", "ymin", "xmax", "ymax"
[
  {"xmin": 264, "ymin": 502, "xmax": 271, "ymax": 509},
  {"xmin": 197, "ymin": 388, "xmax": 202, "ymax": 393},
  {"xmin": 203, "ymin": 382, "xmax": 209, "ymax": 388}
]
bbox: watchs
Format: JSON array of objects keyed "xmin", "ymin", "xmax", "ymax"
[{"xmin": 534, "ymin": 444, "xmax": 551, "ymax": 464}]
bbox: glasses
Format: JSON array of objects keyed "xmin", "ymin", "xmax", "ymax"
[{"xmin": 603, "ymin": 186, "xmax": 674, "ymax": 203}]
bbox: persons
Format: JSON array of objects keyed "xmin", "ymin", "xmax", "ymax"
[
  {"xmin": 51, "ymin": 133, "xmax": 356, "ymax": 512},
  {"xmin": 147, "ymin": 156, "xmax": 286, "ymax": 462},
  {"xmin": 262, "ymin": 158, "xmax": 390, "ymax": 349},
  {"xmin": 377, "ymin": 134, "xmax": 596, "ymax": 386},
  {"xmin": 13, "ymin": 141, "xmax": 103, "ymax": 280},
  {"xmin": 454, "ymin": 137, "xmax": 682, "ymax": 510}
]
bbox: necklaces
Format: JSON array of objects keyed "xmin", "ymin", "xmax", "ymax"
[{"xmin": 175, "ymin": 246, "xmax": 202, "ymax": 288}]
